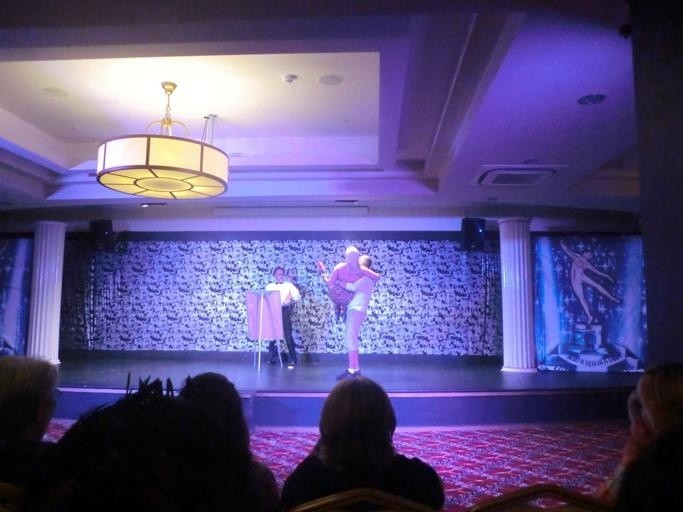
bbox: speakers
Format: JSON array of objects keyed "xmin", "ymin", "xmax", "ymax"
[
  {"xmin": 460, "ymin": 217, "xmax": 486, "ymax": 252},
  {"xmin": 89, "ymin": 218, "xmax": 112, "ymax": 251}
]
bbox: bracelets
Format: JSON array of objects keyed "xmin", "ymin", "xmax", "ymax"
[
  {"xmin": 335, "ymin": 281, "xmax": 340, "ymax": 286},
  {"xmin": 321, "ymin": 275, "xmax": 325, "ymax": 279}
]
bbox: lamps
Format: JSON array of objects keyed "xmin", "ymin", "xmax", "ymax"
[{"xmin": 95, "ymin": 78, "xmax": 232, "ymax": 201}]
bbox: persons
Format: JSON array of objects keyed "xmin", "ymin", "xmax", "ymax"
[
  {"xmin": 588, "ymin": 360, "xmax": 682, "ymax": 511},
  {"xmin": 261, "ymin": 266, "xmax": 302, "ymax": 371},
  {"xmin": 313, "ymin": 246, "xmax": 381, "ymax": 326},
  {"xmin": 1, "ymin": 356, "xmax": 447, "ymax": 510},
  {"xmin": 593, "ymin": 387, "xmax": 657, "ymax": 508},
  {"xmin": 332, "ymin": 254, "xmax": 377, "ymax": 381}
]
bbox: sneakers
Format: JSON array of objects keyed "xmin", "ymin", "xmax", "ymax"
[{"xmin": 337, "ymin": 370, "xmax": 362, "ymax": 381}]
